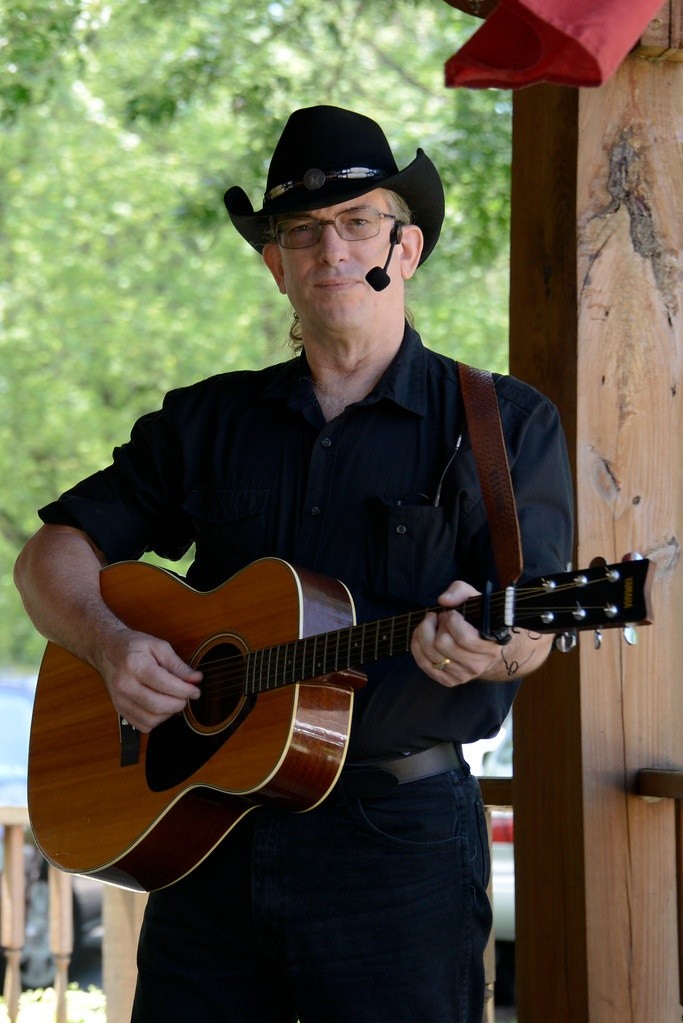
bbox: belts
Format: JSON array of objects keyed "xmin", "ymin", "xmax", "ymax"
[{"xmin": 329, "ymin": 741, "xmax": 463, "ymax": 796}]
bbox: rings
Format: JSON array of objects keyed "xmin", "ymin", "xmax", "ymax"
[{"xmin": 432, "ymin": 659, "xmax": 451, "ymax": 670}]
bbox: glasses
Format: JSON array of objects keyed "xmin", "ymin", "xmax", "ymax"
[{"xmin": 273, "ymin": 207, "xmax": 398, "ymax": 249}]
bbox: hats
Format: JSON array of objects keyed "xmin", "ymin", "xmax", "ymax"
[{"xmin": 223, "ymin": 105, "xmax": 445, "ymax": 269}]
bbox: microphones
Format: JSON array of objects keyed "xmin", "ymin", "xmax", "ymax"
[{"xmin": 366, "ymin": 221, "xmax": 403, "ymax": 292}]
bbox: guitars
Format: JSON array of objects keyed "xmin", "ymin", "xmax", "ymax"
[{"xmin": 26, "ymin": 549, "xmax": 659, "ymax": 896}]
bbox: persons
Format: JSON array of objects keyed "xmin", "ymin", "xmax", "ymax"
[{"xmin": 13, "ymin": 105, "xmax": 575, "ymax": 1023}]
